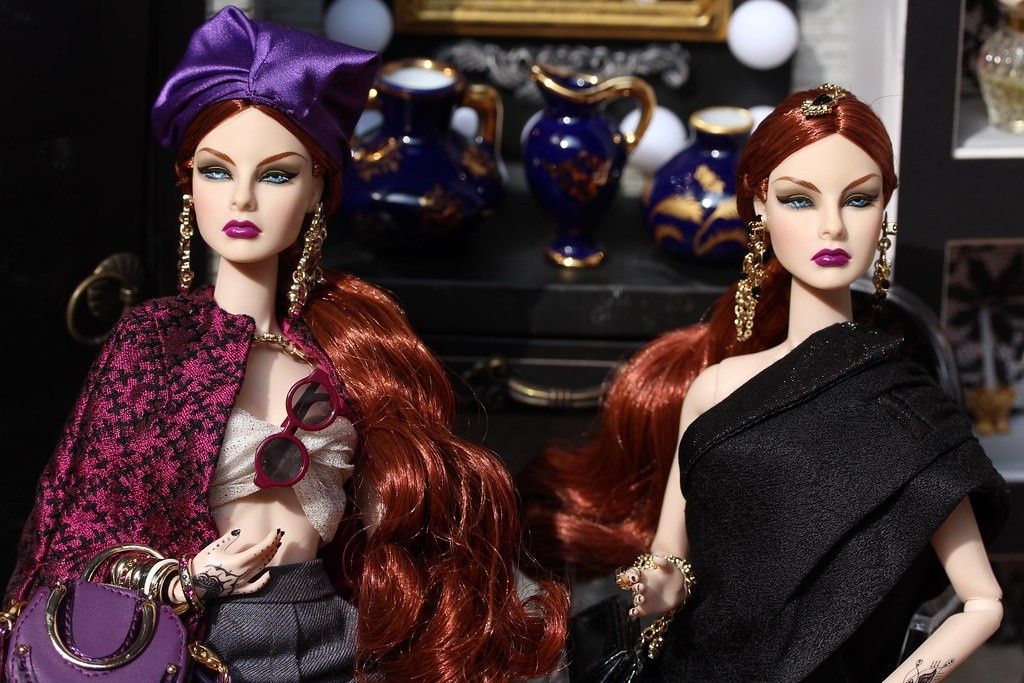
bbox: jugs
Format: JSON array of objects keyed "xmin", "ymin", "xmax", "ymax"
[{"xmin": 522, "ymin": 63, "xmax": 655, "ymax": 265}]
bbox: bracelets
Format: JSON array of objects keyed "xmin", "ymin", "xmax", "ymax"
[{"xmin": 633, "ymin": 553, "xmax": 696, "ymax": 617}]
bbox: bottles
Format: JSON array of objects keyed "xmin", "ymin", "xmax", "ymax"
[
  {"xmin": 975, "ymin": 0, "xmax": 1024, "ymax": 128},
  {"xmin": 346, "ymin": 57, "xmax": 505, "ymax": 279},
  {"xmin": 647, "ymin": 108, "xmax": 754, "ymax": 286}
]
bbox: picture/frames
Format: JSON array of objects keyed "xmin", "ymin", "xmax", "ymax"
[{"xmin": 394, "ymin": 1, "xmax": 732, "ymax": 42}]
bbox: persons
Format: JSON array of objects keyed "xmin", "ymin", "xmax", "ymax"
[
  {"xmin": 14, "ymin": 5, "xmax": 499, "ymax": 683},
  {"xmin": 616, "ymin": 85, "xmax": 1004, "ymax": 683}
]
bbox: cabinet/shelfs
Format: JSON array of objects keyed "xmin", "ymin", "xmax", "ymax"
[
  {"xmin": 321, "ymin": 204, "xmax": 731, "ymax": 491},
  {"xmin": 886, "ymin": 1, "xmax": 1024, "ymax": 647}
]
